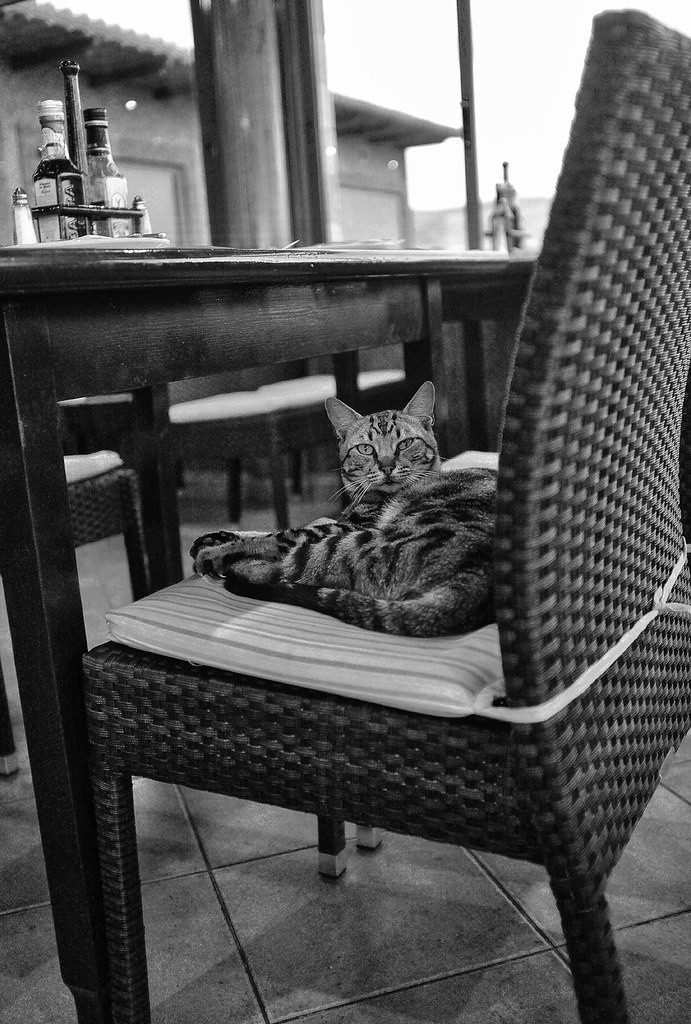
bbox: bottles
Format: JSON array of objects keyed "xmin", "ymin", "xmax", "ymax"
[
  {"xmin": 11, "ymin": 187, "xmax": 39, "ymax": 244},
  {"xmin": 492, "ymin": 199, "xmax": 507, "ymax": 251},
  {"xmin": 83, "ymin": 108, "xmax": 130, "ymax": 237},
  {"xmin": 32, "ymin": 100, "xmax": 88, "ymax": 243},
  {"xmin": 132, "ymin": 195, "xmax": 152, "ymax": 236}
]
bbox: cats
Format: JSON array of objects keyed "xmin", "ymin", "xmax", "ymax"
[{"xmin": 189, "ymin": 381, "xmax": 498, "ymax": 637}]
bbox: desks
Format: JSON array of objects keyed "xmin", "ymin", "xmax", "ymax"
[{"xmin": 0, "ymin": 244, "xmax": 542, "ymax": 1024}]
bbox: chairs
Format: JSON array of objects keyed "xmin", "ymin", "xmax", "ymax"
[
  {"xmin": 78, "ymin": 7, "xmax": 691, "ymax": 1024},
  {"xmin": 61, "ymin": 349, "xmax": 409, "ymax": 600}
]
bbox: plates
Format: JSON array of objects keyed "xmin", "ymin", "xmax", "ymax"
[{"xmin": 304, "ymin": 239, "xmax": 406, "ymax": 253}]
